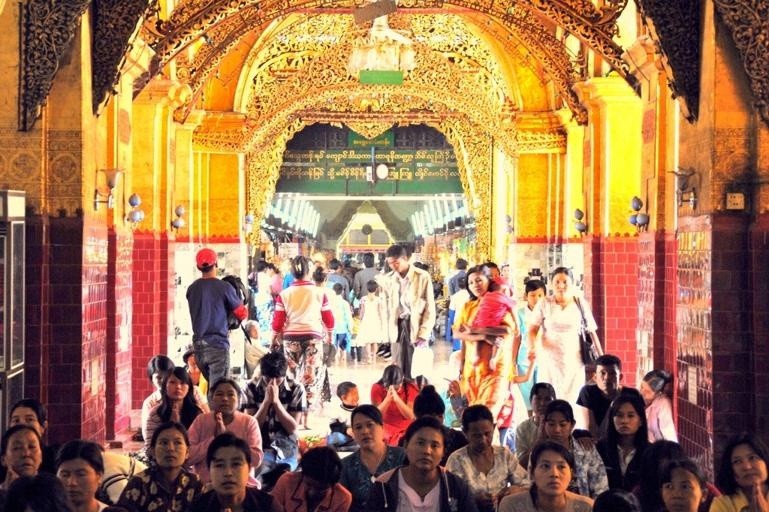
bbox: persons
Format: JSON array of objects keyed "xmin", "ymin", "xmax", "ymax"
[{"xmin": 0, "ymin": 246, "xmax": 768, "ymax": 511}]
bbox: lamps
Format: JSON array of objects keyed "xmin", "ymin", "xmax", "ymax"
[
  {"xmin": 574, "ymin": 191, "xmax": 589, "ymax": 236},
  {"xmin": 168, "ymin": 191, "xmax": 186, "ymax": 235},
  {"xmin": 119, "ymin": 189, "xmax": 144, "ymax": 228},
  {"xmin": 628, "ymin": 178, "xmax": 652, "ymax": 235},
  {"xmin": 347, "ymin": 11, "xmax": 418, "ymax": 87},
  {"xmin": 91, "ymin": 165, "xmax": 127, "ymax": 212},
  {"xmin": 665, "ymin": 162, "xmax": 701, "ymax": 214},
  {"xmin": 503, "ymin": 213, "xmax": 514, "ymax": 236},
  {"xmin": 240, "ymin": 212, "xmax": 256, "ymax": 235}
]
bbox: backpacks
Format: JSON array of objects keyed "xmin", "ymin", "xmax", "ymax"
[{"xmin": 222, "ymin": 275, "xmax": 248, "ymax": 330}]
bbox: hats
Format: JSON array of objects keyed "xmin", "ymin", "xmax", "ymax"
[{"xmin": 197, "ymin": 249, "xmax": 216, "ymax": 268}]
